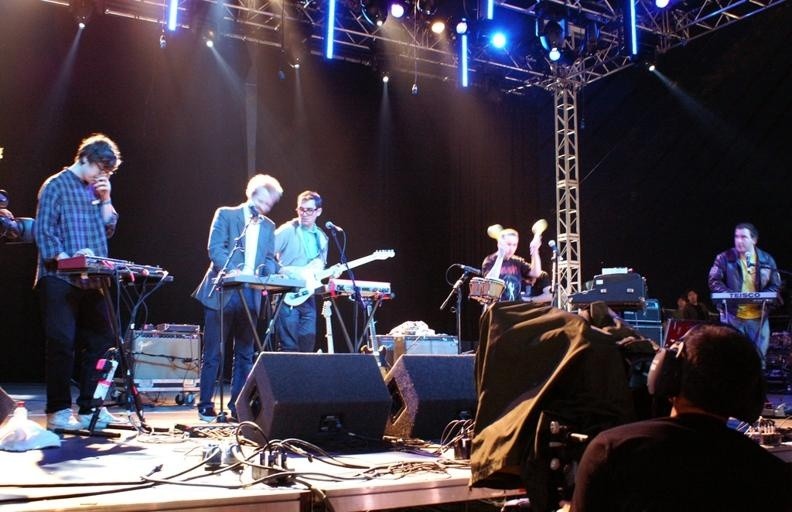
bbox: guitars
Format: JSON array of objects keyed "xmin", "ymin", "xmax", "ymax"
[{"xmin": 278, "ymin": 249, "xmax": 395, "ymax": 306}]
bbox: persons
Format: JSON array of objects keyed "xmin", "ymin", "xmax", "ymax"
[
  {"xmin": 711, "ymin": 222, "xmax": 783, "ymax": 371},
  {"xmin": 32, "ymin": 131, "xmax": 123, "ymax": 432},
  {"xmin": 675, "ymin": 288, "xmax": 710, "ymax": 321},
  {"xmin": 0, "ymin": 189, "xmax": 22, "ymax": 243},
  {"xmin": 480, "ymin": 223, "xmax": 544, "ymax": 303},
  {"xmin": 569, "ymin": 321, "xmax": 792, "ymax": 510},
  {"xmin": 190, "ymin": 172, "xmax": 284, "ymax": 423},
  {"xmin": 271, "ymin": 190, "xmax": 329, "ymax": 354}
]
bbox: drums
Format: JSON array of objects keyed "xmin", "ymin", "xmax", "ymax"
[
  {"xmin": 481, "ymin": 278, "xmax": 506, "ymax": 300},
  {"xmin": 469, "ymin": 276, "xmax": 486, "ymax": 297}
]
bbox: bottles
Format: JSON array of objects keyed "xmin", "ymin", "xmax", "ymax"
[{"xmin": 13, "ymin": 401, "xmax": 28, "ymax": 439}]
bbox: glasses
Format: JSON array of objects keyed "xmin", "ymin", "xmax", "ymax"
[
  {"xmin": 94, "ymin": 163, "xmax": 113, "ymax": 176},
  {"xmin": 295, "ymin": 207, "xmax": 317, "ymax": 216}
]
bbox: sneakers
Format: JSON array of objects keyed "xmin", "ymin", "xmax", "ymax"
[
  {"xmin": 46, "ymin": 407, "xmax": 83, "ymax": 430},
  {"xmin": 197, "ymin": 408, "xmax": 217, "ymax": 422},
  {"xmin": 76, "ymin": 407, "xmax": 127, "ymax": 429}
]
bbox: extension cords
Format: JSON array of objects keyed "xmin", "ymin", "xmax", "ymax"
[{"xmin": 245, "ymin": 464, "xmax": 294, "ymax": 486}]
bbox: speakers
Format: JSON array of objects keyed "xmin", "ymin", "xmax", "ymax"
[
  {"xmin": 235, "ymin": 351, "xmax": 392, "ymax": 452},
  {"xmin": 383, "ymin": 354, "xmax": 477, "ymax": 441},
  {"xmin": 128, "ymin": 324, "xmax": 202, "ymax": 380},
  {"xmin": 369, "ymin": 335, "xmax": 459, "ymax": 379}
]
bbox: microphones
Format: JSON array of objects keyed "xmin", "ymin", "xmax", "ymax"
[
  {"xmin": 548, "ymin": 240, "xmax": 563, "ymax": 261},
  {"xmin": 461, "ymin": 264, "xmax": 481, "ymax": 275},
  {"xmin": 745, "ymin": 251, "xmax": 751, "ymax": 274},
  {"xmin": 249, "ymin": 202, "xmax": 265, "ymax": 224},
  {"xmin": 326, "ymin": 221, "xmax": 343, "ymax": 233}
]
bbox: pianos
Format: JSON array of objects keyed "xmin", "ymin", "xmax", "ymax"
[
  {"xmin": 567, "ymin": 272, "xmax": 648, "ymax": 312},
  {"xmin": 212, "ymin": 274, "xmax": 307, "ymax": 293},
  {"xmin": 314, "ymin": 278, "xmax": 391, "ymax": 298},
  {"xmin": 58, "ymin": 254, "xmax": 174, "ymax": 286},
  {"xmin": 710, "ymin": 291, "xmax": 784, "ymax": 306}
]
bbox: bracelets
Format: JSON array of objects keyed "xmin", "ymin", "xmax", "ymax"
[{"xmin": 101, "ymin": 200, "xmax": 111, "ymax": 207}]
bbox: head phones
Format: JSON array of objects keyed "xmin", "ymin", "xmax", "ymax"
[{"xmin": 647, "ymin": 321, "xmax": 765, "ymax": 425}]
